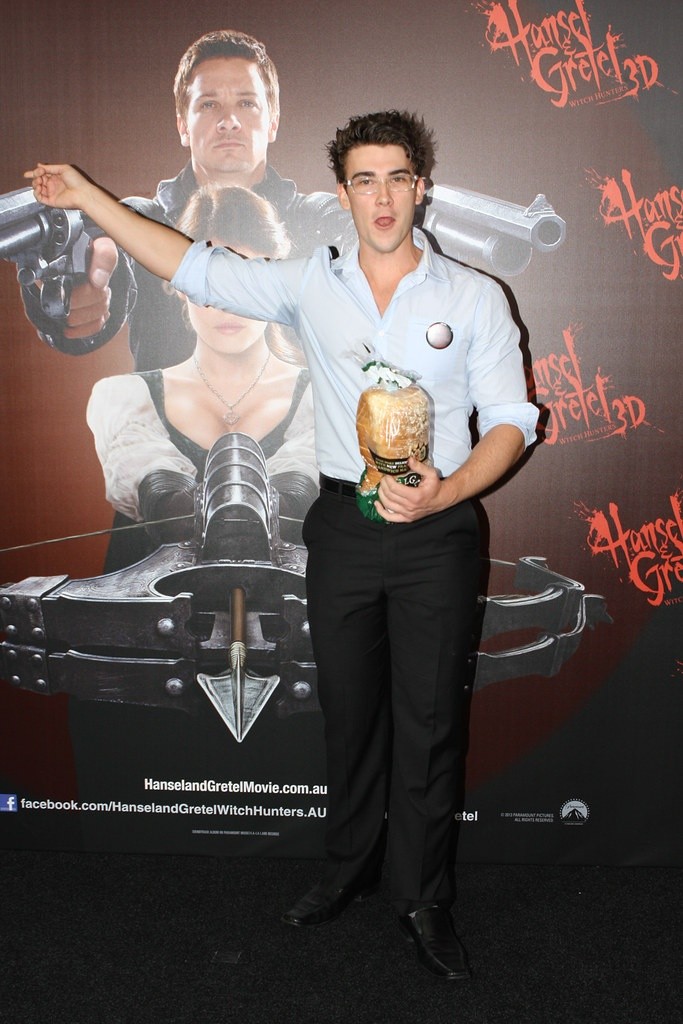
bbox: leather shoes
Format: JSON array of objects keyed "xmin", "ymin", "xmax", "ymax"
[
  {"xmin": 397, "ymin": 907, "xmax": 470, "ymax": 980},
  {"xmin": 282, "ymin": 876, "xmax": 382, "ymax": 928}
]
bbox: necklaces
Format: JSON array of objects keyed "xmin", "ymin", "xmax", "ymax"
[{"xmin": 191, "ymin": 350, "xmax": 270, "ymax": 426}]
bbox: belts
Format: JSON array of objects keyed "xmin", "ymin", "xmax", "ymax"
[{"xmin": 319, "ymin": 471, "xmax": 356, "ymax": 499}]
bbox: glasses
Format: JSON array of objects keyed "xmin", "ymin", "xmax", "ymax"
[{"xmin": 342, "ymin": 175, "xmax": 420, "ymax": 195}]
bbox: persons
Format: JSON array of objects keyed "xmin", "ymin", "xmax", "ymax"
[
  {"xmin": 33, "ymin": 29, "xmax": 358, "ymax": 575},
  {"xmin": 23, "ymin": 110, "xmax": 540, "ymax": 981}
]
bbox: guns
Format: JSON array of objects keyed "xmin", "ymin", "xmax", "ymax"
[
  {"xmin": 0, "ymin": 185, "xmax": 94, "ymax": 321},
  {"xmin": 415, "ymin": 181, "xmax": 567, "ymax": 277}
]
